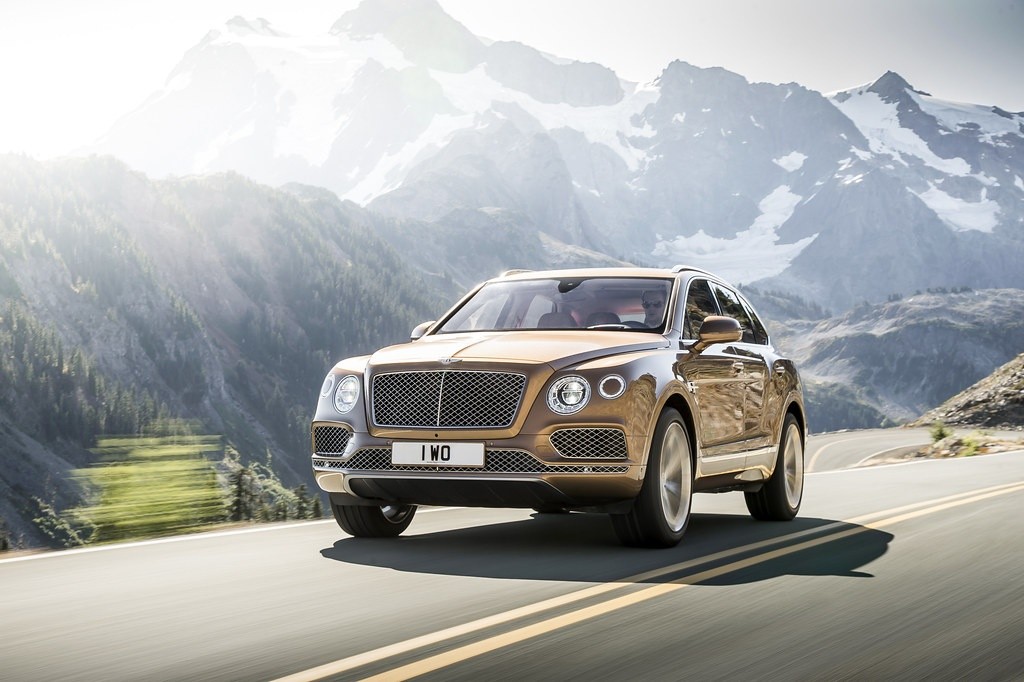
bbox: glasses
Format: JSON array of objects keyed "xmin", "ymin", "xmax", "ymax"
[{"xmin": 641, "ymin": 299, "xmax": 664, "ymax": 309}]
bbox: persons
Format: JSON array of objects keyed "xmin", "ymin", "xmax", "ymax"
[{"xmin": 637, "ymin": 287, "xmax": 671, "ymax": 331}]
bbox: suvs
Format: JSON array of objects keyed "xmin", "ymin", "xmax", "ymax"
[{"xmin": 309, "ymin": 267, "xmax": 806, "ymax": 548}]
